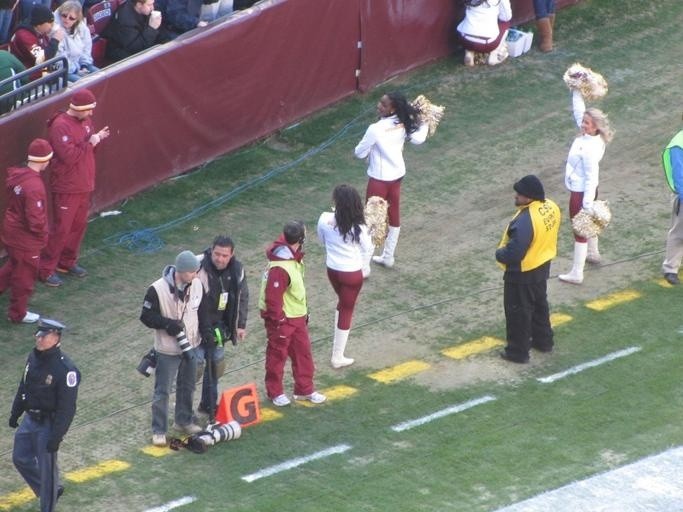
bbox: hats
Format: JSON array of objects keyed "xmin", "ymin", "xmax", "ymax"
[
  {"xmin": 512, "ymin": 175, "xmax": 544, "ymax": 200},
  {"xmin": 175, "ymin": 250, "xmax": 201, "ymax": 273},
  {"xmin": 34, "ymin": 318, "xmax": 65, "ymax": 336},
  {"xmin": 30, "ymin": 3, "xmax": 53, "ymax": 24},
  {"xmin": 26, "ymin": 138, "xmax": 54, "ymax": 163},
  {"xmin": 68, "ymin": 88, "xmax": 97, "ymax": 111}
]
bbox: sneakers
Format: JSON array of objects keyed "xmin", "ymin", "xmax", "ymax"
[
  {"xmin": 171, "ymin": 420, "xmax": 203, "ymax": 435},
  {"xmin": 19, "ymin": 261, "xmax": 93, "ymax": 327},
  {"xmin": 152, "ymin": 433, "xmax": 168, "ymax": 447},
  {"xmin": 293, "ymin": 389, "xmax": 328, "ymax": 405},
  {"xmin": 497, "ymin": 347, "xmax": 531, "ymax": 364},
  {"xmin": 270, "ymin": 392, "xmax": 292, "ymax": 408}
]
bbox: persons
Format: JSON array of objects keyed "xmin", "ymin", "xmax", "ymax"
[
  {"xmin": 46, "ymin": 89, "xmax": 111, "ymax": 290},
  {"xmin": 493, "ymin": 172, "xmax": 565, "ymax": 364},
  {"xmin": 5, "ymin": 318, "xmax": 83, "ymax": 507},
  {"xmin": 661, "ymin": 113, "xmax": 683, "ymax": 286},
  {"xmin": 0, "ymin": 139, "xmax": 56, "ymax": 324},
  {"xmin": 452, "ymin": 0, "xmax": 513, "ymax": 66},
  {"xmin": 559, "ymin": 84, "xmax": 616, "ymax": 287},
  {"xmin": 531, "ymin": 1, "xmax": 560, "ymax": 54},
  {"xmin": 0, "ymin": 1, "xmax": 264, "ymax": 120},
  {"xmin": 355, "ymin": 90, "xmax": 432, "ymax": 267}
]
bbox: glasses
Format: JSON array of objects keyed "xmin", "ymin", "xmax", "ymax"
[{"xmin": 60, "ymin": 13, "xmax": 79, "ymax": 22}]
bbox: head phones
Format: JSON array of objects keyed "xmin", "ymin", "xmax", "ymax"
[{"xmin": 291, "ymin": 220, "xmax": 305, "ymax": 244}]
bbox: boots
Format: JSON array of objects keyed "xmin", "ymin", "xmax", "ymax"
[
  {"xmin": 556, "ymin": 239, "xmax": 590, "ymax": 288},
  {"xmin": 330, "ymin": 321, "xmax": 356, "ymax": 370},
  {"xmin": 368, "ymin": 223, "xmax": 401, "ymax": 270},
  {"xmin": 460, "ymin": 12, "xmax": 558, "ymax": 67},
  {"xmin": 583, "ymin": 235, "xmax": 605, "ymax": 265}
]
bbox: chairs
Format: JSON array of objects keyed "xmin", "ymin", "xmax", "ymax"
[{"xmin": 0, "ymin": 0, "xmax": 125, "ymax": 86}]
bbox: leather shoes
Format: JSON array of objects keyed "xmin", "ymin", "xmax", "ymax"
[{"xmin": 661, "ymin": 263, "xmax": 682, "ymax": 286}]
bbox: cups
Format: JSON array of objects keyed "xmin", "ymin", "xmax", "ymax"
[{"xmin": 151, "ymin": 11, "xmax": 162, "ymax": 18}]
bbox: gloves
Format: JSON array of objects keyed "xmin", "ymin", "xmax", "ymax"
[
  {"xmin": 164, "ymin": 317, "xmax": 185, "ymax": 336},
  {"xmin": 8, "ymin": 415, "xmax": 20, "ymax": 428},
  {"xmin": 43, "ymin": 433, "xmax": 64, "ymax": 453}
]
